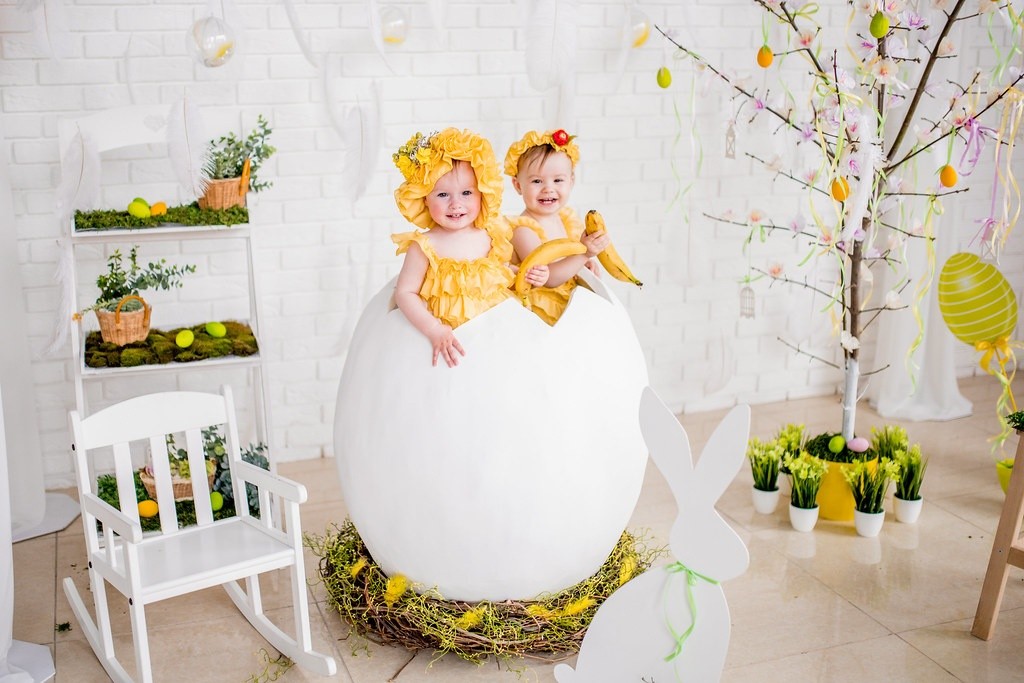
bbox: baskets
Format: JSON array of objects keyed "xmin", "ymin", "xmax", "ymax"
[
  {"xmin": 94, "ymin": 295, "xmax": 153, "ymax": 347},
  {"xmin": 195, "ymin": 157, "xmax": 251, "ymax": 212},
  {"xmin": 139, "ymin": 457, "xmax": 218, "ymax": 503}
]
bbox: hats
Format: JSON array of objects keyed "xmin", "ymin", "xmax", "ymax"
[
  {"xmin": 392, "ymin": 125, "xmax": 505, "ymax": 230},
  {"xmin": 503, "ymin": 127, "xmax": 581, "ymax": 177}
]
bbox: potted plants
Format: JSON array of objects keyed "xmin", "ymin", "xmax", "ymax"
[{"xmin": 805, "ymin": 431, "xmax": 880, "ymax": 522}]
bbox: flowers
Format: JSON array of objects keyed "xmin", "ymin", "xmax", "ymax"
[
  {"xmin": 782, "ymin": 449, "xmax": 830, "ymax": 508},
  {"xmin": 839, "ymin": 453, "xmax": 901, "ymax": 513},
  {"xmin": 871, "ymin": 423, "xmax": 909, "ymax": 463},
  {"xmin": 894, "ymin": 442, "xmax": 929, "ymax": 497},
  {"xmin": 774, "ymin": 422, "xmax": 811, "ymax": 473},
  {"xmin": 747, "ymin": 435, "xmax": 785, "ymax": 490}
]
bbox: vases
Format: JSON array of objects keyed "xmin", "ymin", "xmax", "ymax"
[
  {"xmin": 789, "ymin": 502, "xmax": 820, "ymax": 533},
  {"xmin": 893, "ymin": 494, "xmax": 924, "ymax": 524},
  {"xmin": 884, "ymin": 477, "xmax": 897, "ymax": 500},
  {"xmin": 751, "ymin": 484, "xmax": 781, "ymax": 515},
  {"xmin": 778, "ymin": 473, "xmax": 793, "ymax": 497},
  {"xmin": 854, "ymin": 506, "xmax": 885, "ymax": 538}
]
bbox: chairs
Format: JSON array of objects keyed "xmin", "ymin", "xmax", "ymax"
[{"xmin": 60, "ymin": 382, "xmax": 336, "ymax": 683}]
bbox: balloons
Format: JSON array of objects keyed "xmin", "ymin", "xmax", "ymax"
[{"xmin": 938, "ymin": 254, "xmax": 1019, "ymax": 351}]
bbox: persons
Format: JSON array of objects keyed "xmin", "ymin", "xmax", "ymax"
[
  {"xmin": 504, "ymin": 130, "xmax": 610, "ymax": 328},
  {"xmin": 389, "ymin": 128, "xmax": 550, "ymax": 368}
]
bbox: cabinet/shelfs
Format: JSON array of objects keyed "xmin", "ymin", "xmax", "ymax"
[{"xmin": 66, "ymin": 210, "xmax": 283, "ymax": 533}]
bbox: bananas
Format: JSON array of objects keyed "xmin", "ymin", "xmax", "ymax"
[
  {"xmin": 515, "ymin": 238, "xmax": 587, "ymax": 307},
  {"xmin": 585, "ymin": 209, "xmax": 643, "ymax": 286}
]
